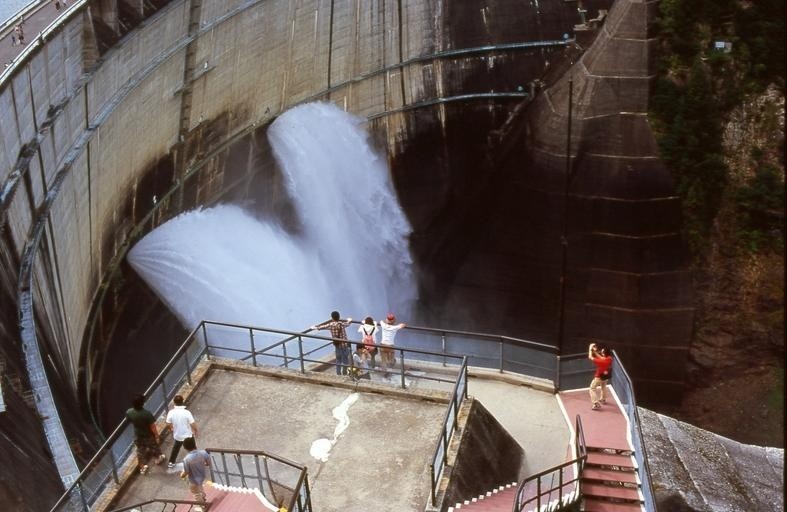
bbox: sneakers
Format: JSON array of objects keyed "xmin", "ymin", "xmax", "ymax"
[
  {"xmin": 168, "ymin": 461, "xmax": 176, "ymax": 468},
  {"xmin": 155, "ymin": 454, "xmax": 165, "ymax": 465},
  {"xmin": 140, "ymin": 465, "xmax": 149, "ymax": 475},
  {"xmin": 592, "ymin": 404, "xmax": 601, "ymax": 409},
  {"xmin": 599, "ymin": 399, "xmax": 605, "ymax": 402}
]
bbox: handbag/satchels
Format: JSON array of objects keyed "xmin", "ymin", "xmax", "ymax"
[
  {"xmin": 600, "ymin": 369, "xmax": 611, "ymax": 380},
  {"xmin": 333, "ymin": 338, "xmax": 339, "ymax": 345}
]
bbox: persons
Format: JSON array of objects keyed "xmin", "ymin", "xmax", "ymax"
[
  {"xmin": 176, "ymin": 438, "xmax": 214, "ymax": 512},
  {"xmin": 309, "ymin": 309, "xmax": 409, "ymax": 380},
  {"xmin": 125, "ymin": 394, "xmax": 166, "ymax": 476},
  {"xmin": 586, "ymin": 341, "xmax": 613, "ymax": 410},
  {"xmin": 162, "ymin": 395, "xmax": 203, "ymax": 467}
]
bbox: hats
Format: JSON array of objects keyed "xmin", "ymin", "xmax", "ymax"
[{"xmin": 387, "ymin": 314, "xmax": 394, "ymax": 321}]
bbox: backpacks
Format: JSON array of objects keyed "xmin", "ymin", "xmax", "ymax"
[{"xmin": 363, "ymin": 327, "xmax": 375, "ymax": 352}]
